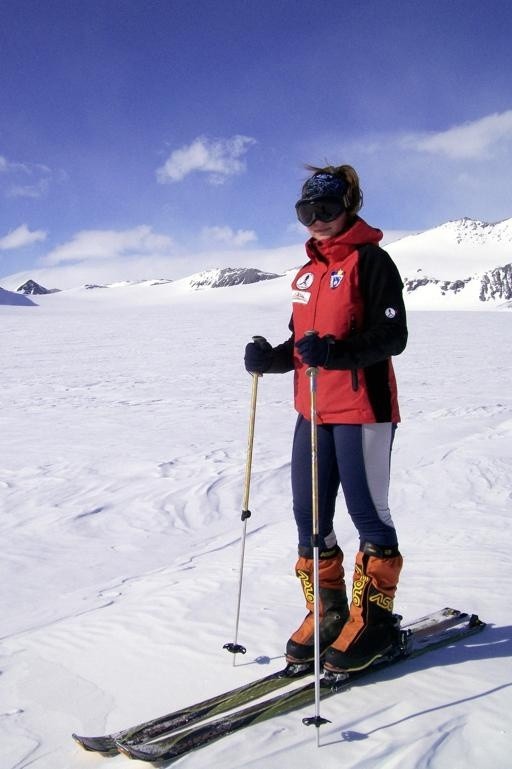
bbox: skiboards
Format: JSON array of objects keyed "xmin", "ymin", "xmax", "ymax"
[{"xmin": 72, "ymin": 606, "xmax": 490, "ymax": 765}]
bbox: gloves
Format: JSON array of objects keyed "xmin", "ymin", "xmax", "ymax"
[
  {"xmin": 244, "ymin": 336, "xmax": 274, "ymax": 372},
  {"xmin": 296, "ymin": 334, "xmax": 326, "ymax": 366}
]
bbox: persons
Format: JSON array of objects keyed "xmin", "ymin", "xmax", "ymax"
[{"xmin": 243, "ymin": 163, "xmax": 408, "ymax": 674}]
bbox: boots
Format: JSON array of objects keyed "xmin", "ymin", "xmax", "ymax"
[
  {"xmin": 286, "ymin": 544, "xmax": 349, "ymax": 663},
  {"xmin": 323, "ymin": 541, "xmax": 402, "ymax": 672}
]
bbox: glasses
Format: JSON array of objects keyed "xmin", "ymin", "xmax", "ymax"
[{"xmin": 295, "ymin": 195, "xmax": 344, "ymax": 227}]
